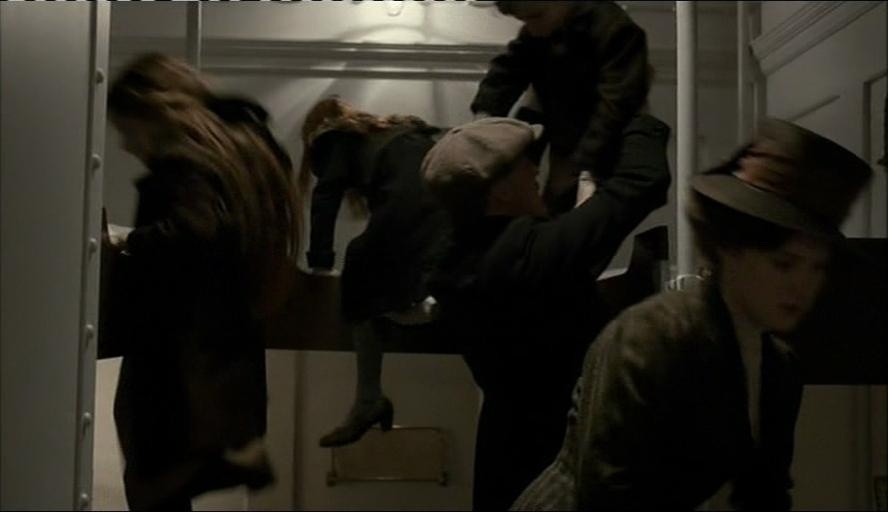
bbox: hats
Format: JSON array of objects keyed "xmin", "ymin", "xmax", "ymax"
[
  {"xmin": 687, "ymin": 112, "xmax": 875, "ymax": 248},
  {"xmin": 420, "ymin": 117, "xmax": 546, "ymax": 204}
]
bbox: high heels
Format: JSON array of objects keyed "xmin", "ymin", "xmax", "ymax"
[{"xmin": 317, "ymin": 397, "xmax": 396, "ymax": 449}]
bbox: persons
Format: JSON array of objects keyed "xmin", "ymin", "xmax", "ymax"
[
  {"xmin": 469, "ymin": 1, "xmax": 654, "ymax": 215},
  {"xmin": 98, "ymin": 49, "xmax": 304, "ymax": 511},
  {"xmin": 507, "ymin": 115, "xmax": 877, "ymax": 512},
  {"xmin": 419, "ymin": 114, "xmax": 669, "ymax": 511},
  {"xmin": 299, "ymin": 93, "xmax": 454, "ymax": 449}
]
